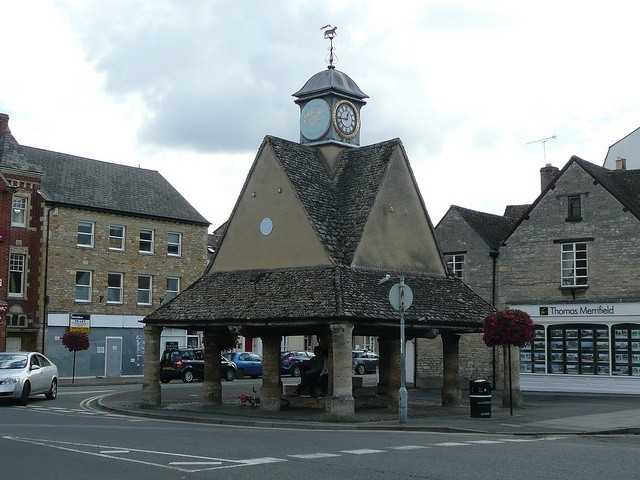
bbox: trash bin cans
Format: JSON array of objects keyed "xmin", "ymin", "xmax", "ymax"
[{"xmin": 470, "ymin": 379, "xmax": 492, "ymax": 417}]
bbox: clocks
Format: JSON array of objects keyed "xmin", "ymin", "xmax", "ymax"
[{"xmin": 332, "ymin": 100, "xmax": 361, "ymax": 139}]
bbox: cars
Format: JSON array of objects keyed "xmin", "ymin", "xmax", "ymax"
[
  {"xmin": 160, "ymin": 347, "xmax": 239, "ymax": 384},
  {"xmin": 280, "ymin": 350, "xmax": 316, "ymax": 377},
  {"xmin": 222, "ymin": 352, "xmax": 263, "ymax": 379},
  {"xmin": 1, "ymin": 350, "xmax": 59, "ymax": 405},
  {"xmin": 351, "ymin": 348, "xmax": 380, "ymax": 373}
]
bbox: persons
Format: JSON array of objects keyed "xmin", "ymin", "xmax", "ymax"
[
  {"xmin": 290, "ymin": 346, "xmax": 323, "ymax": 398},
  {"xmin": 317, "ymin": 350, "xmax": 329, "ymax": 398}
]
